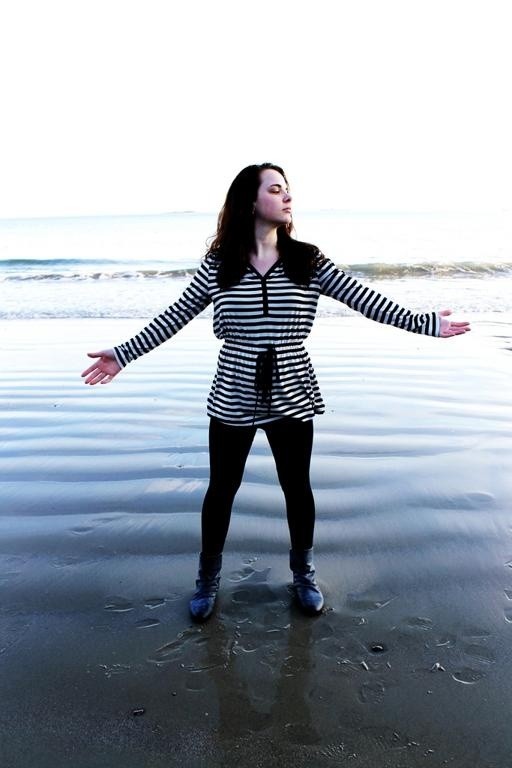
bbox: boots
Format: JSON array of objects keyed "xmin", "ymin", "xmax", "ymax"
[
  {"xmin": 188, "ymin": 552, "xmax": 223, "ymax": 622},
  {"xmin": 289, "ymin": 546, "xmax": 324, "ymax": 616}
]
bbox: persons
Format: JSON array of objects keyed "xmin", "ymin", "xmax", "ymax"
[{"xmin": 80, "ymin": 162, "xmax": 473, "ymax": 620}]
